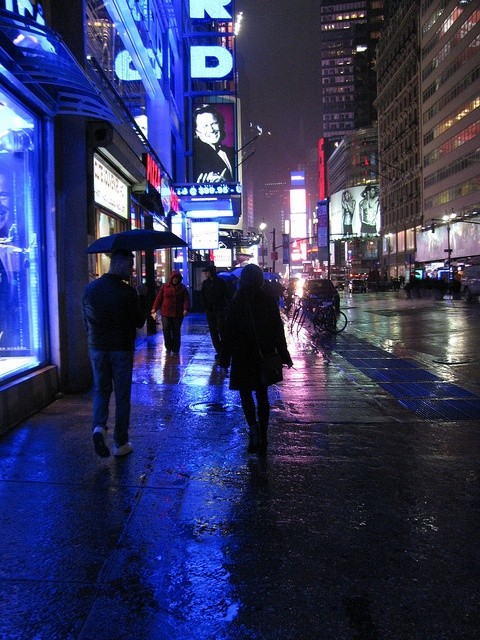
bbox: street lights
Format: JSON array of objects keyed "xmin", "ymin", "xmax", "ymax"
[
  {"xmin": 443, "ymin": 207, "xmax": 457, "ymax": 285},
  {"xmin": 258, "ymin": 216, "xmax": 266, "ymax": 270}
]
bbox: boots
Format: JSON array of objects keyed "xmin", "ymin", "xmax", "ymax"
[
  {"xmin": 260, "ymin": 425, "xmax": 268, "ymax": 452},
  {"xmin": 247, "ymin": 421, "xmax": 262, "ymax": 458}
]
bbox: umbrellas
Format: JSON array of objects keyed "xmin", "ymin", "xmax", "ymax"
[
  {"xmin": 410, "ymin": 271, "xmax": 420, "ymax": 275},
  {"xmin": 263, "ymin": 271, "xmax": 282, "ymax": 280},
  {"xmin": 84, "ymin": 227, "xmax": 190, "ymax": 295},
  {"xmin": 217, "ymin": 271, "xmax": 237, "ymax": 281},
  {"xmin": 231, "ymin": 266, "xmax": 245, "ymax": 281}
]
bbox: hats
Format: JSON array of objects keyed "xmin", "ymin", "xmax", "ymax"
[
  {"xmin": 201, "ymin": 265, "xmax": 216, "ymax": 272},
  {"xmin": 240, "ymin": 263, "xmax": 263, "ymax": 287}
]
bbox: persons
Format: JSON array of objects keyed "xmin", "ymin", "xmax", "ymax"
[
  {"xmin": 80, "ymin": 252, "xmax": 149, "ymax": 460},
  {"xmin": 405, "ymin": 276, "xmax": 461, "ymax": 299},
  {"xmin": 128, "ymin": 109, "xmax": 148, "ymax": 141},
  {"xmin": 263, "ymin": 279, "xmax": 283, "ymax": 310},
  {"xmin": 0, "ymin": 168, "xmax": 23, "ymax": 350},
  {"xmin": 217, "ymin": 263, "xmax": 293, "ymax": 455},
  {"xmin": 150, "ymin": 271, "xmax": 190, "ymax": 355},
  {"xmin": 358, "ymin": 185, "xmax": 380, "ymax": 238},
  {"xmin": 190, "ymin": 102, "xmax": 234, "ymax": 183},
  {"xmin": 340, "ymin": 188, "xmax": 356, "ymax": 236},
  {"xmin": 199, "ymin": 263, "xmax": 230, "ymax": 365}
]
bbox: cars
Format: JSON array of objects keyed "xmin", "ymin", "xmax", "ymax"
[
  {"xmin": 303, "ymin": 279, "xmax": 340, "ymax": 309},
  {"xmin": 348, "ymin": 278, "xmax": 367, "ymax": 293},
  {"xmin": 331, "ymin": 275, "xmax": 345, "ymax": 289}
]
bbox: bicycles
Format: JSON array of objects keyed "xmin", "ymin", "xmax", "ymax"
[
  {"xmin": 296, "ymin": 290, "xmax": 347, "ymax": 334},
  {"xmin": 290, "ymin": 293, "xmax": 337, "ymax": 334}
]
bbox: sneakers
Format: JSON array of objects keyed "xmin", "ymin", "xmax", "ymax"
[
  {"xmin": 173, "ymin": 350, "xmax": 179, "ymax": 355},
  {"xmin": 114, "ymin": 441, "xmax": 134, "ymax": 456},
  {"xmin": 165, "ymin": 347, "xmax": 173, "ymax": 353},
  {"xmin": 94, "ymin": 425, "xmax": 111, "ymax": 457}
]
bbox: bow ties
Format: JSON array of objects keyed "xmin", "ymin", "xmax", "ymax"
[{"xmin": 215, "ymin": 143, "xmax": 227, "ymax": 154}]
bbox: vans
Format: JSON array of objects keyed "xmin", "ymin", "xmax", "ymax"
[{"xmin": 459, "ymin": 256, "xmax": 480, "ymax": 302}]
